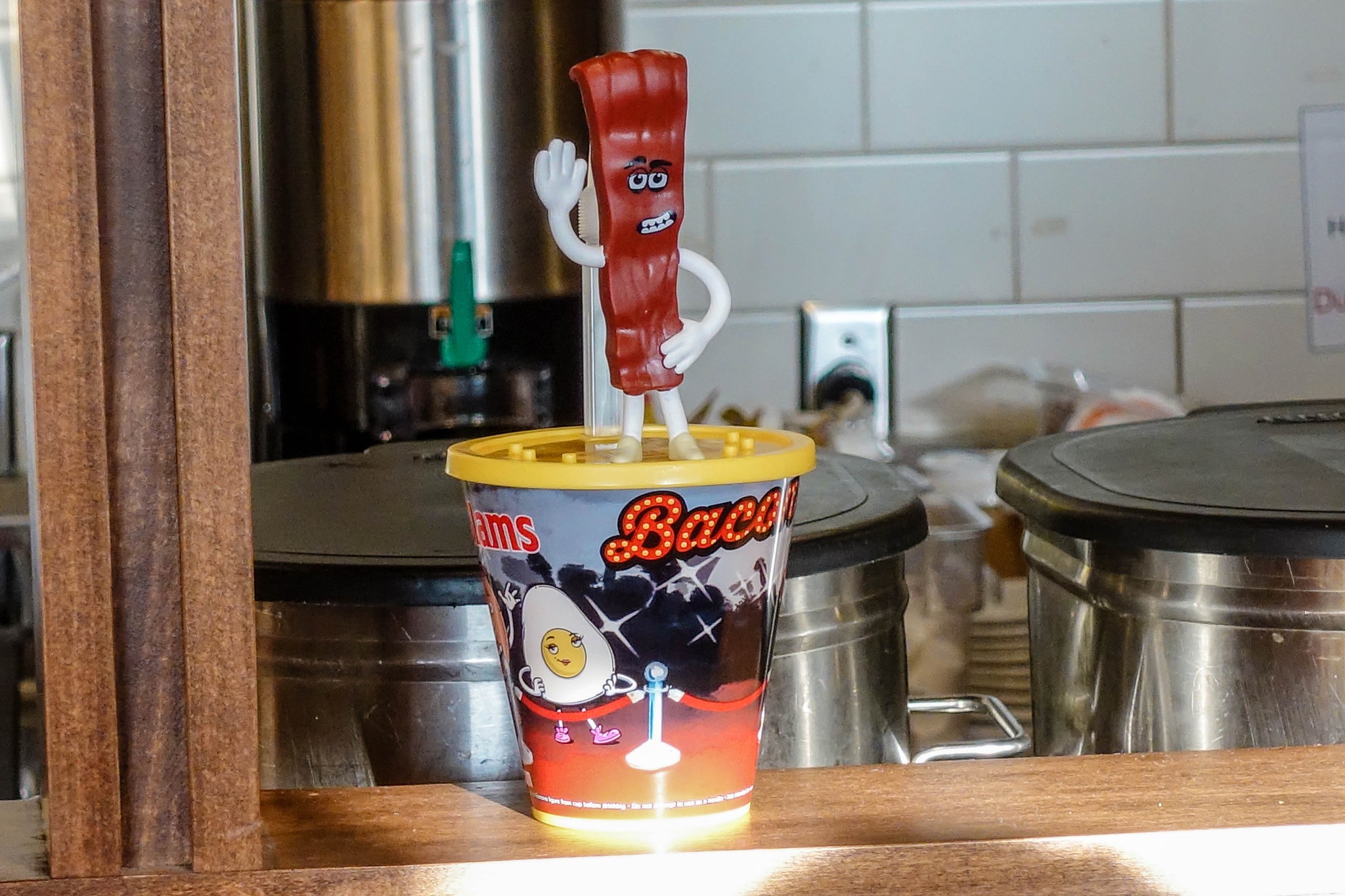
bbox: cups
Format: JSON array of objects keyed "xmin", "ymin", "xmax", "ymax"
[
  {"xmin": 448, "ymin": 423, "xmax": 817, "ymax": 848},
  {"xmin": 899, "ymin": 513, "xmax": 992, "ymax": 746}
]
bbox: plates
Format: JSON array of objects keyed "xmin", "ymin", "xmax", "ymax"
[{"xmin": 967, "ymin": 578, "xmax": 1035, "ymax": 742}]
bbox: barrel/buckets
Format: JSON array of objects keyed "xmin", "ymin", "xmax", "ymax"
[
  {"xmin": 257, "ymin": 440, "xmax": 1031, "ymax": 791},
  {"xmin": 262, "ymin": 1, "xmax": 577, "ymax": 304},
  {"xmin": 996, "ymin": 399, "xmax": 1345, "ymax": 758}
]
